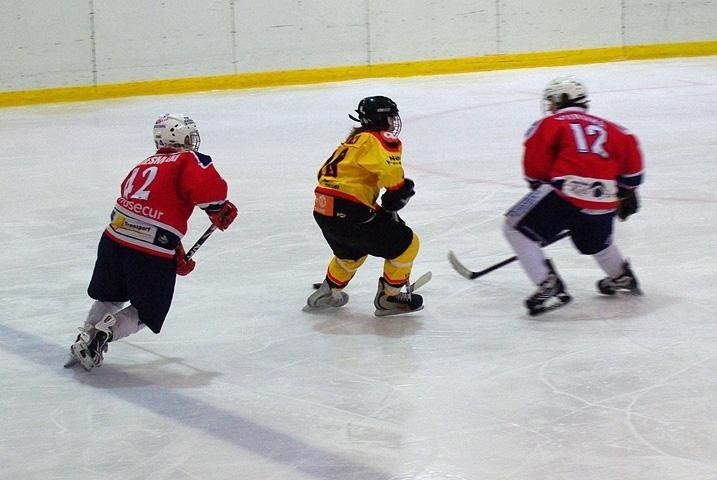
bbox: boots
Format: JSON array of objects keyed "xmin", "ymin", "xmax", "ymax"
[
  {"xmin": 373, "ymin": 277, "xmax": 422, "ymax": 310},
  {"xmin": 526, "ymin": 259, "xmax": 565, "ymax": 309},
  {"xmin": 70, "ymin": 313, "xmax": 116, "ymax": 368},
  {"xmin": 306, "ymin": 279, "xmax": 348, "ymax": 308},
  {"xmin": 598, "ymin": 262, "xmax": 638, "ymax": 292}
]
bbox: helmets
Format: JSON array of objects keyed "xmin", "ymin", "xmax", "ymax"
[
  {"xmin": 539, "ymin": 74, "xmax": 589, "ymax": 114},
  {"xmin": 152, "ymin": 112, "xmax": 200, "ymax": 152},
  {"xmin": 348, "ymin": 95, "xmax": 401, "ymax": 137}
]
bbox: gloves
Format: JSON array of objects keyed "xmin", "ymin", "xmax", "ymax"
[
  {"xmin": 615, "ymin": 188, "xmax": 638, "ymax": 222},
  {"xmin": 381, "ymin": 178, "xmax": 415, "ymax": 211},
  {"xmin": 174, "ymin": 240, "xmax": 195, "ymax": 276},
  {"xmin": 531, "ymin": 181, "xmax": 541, "ymax": 191},
  {"xmin": 205, "ymin": 200, "xmax": 237, "ymax": 231}
]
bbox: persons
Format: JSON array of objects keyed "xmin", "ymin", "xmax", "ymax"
[
  {"xmin": 308, "ymin": 95, "xmax": 423, "ymax": 311},
  {"xmin": 503, "ymin": 72, "xmax": 647, "ymax": 314},
  {"xmin": 67, "ymin": 114, "xmax": 236, "ymax": 371}
]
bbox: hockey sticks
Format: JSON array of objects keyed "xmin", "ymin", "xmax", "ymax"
[
  {"xmin": 448, "ymin": 231, "xmax": 573, "ymax": 279},
  {"xmin": 391, "ymin": 209, "xmax": 432, "ymax": 293}
]
